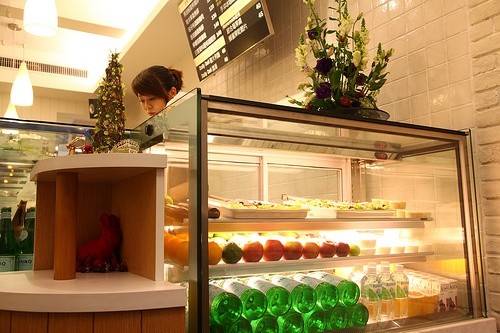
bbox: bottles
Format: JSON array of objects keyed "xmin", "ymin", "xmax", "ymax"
[
  {"xmin": 238, "ymin": 274, "xmax": 317, "ymax": 317},
  {"xmin": 391, "ymin": 265, "xmax": 409, "ymax": 320},
  {"xmin": 321, "ymin": 306, "xmax": 348, "ymax": 331},
  {"xmin": 224, "ymin": 316, "xmax": 253, "ymax": 333},
  {"xmin": 271, "ymin": 309, "xmax": 304, "ymax": 333},
  {"xmin": 242, "ymin": 310, "xmax": 279, "ymax": 333},
  {"xmin": 360, "ymin": 268, "xmax": 382, "ymax": 324},
  {"xmin": 379, "ymin": 267, "xmax": 395, "ymax": 323},
  {"xmin": 287, "ymin": 271, "xmax": 360, "ymax": 314},
  {"xmin": 209, "ymin": 278, "xmax": 268, "ymax": 325},
  {"xmin": 299, "ymin": 306, "xmax": 327, "ymax": 333},
  {"xmin": 15, "ymin": 207, "xmax": 36, "ymax": 271},
  {"xmin": 348, "ymin": 260, "xmax": 390, "ymax": 285},
  {"xmin": 0, "ymin": 207, "xmax": 16, "ymax": 272},
  {"xmin": 343, "ymin": 303, "xmax": 369, "ymax": 328}
]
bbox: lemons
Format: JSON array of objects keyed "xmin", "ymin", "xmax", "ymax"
[
  {"xmin": 164, "ymin": 195, "xmax": 174, "ymax": 205},
  {"xmin": 350, "ymin": 245, "xmax": 360, "ymax": 255}
]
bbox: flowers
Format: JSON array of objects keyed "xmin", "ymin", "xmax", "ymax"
[{"xmin": 283, "ymin": 0, "xmax": 395, "ymax": 109}]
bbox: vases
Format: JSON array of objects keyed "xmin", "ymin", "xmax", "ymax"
[{"xmin": 324, "ymin": 108, "xmax": 390, "ymax": 121}]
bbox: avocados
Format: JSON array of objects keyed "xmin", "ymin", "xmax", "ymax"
[{"xmin": 221, "ymin": 242, "xmax": 242, "ymax": 264}]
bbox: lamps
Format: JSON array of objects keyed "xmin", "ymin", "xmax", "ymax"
[
  {"xmin": 10, "ymin": 32, "xmax": 34, "ymax": 106},
  {"xmin": 23, "ymin": 0, "xmax": 58, "ymax": 37},
  {"xmin": 1, "ymin": 23, "xmax": 22, "ymax": 134}
]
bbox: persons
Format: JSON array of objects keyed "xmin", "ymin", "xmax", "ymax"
[{"xmin": 131, "ymin": 65, "xmax": 189, "ymax": 202}]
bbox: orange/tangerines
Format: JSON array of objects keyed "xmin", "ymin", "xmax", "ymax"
[{"xmin": 164, "ymin": 234, "xmax": 223, "ymax": 266}]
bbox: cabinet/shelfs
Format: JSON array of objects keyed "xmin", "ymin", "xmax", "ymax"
[{"xmin": 127, "ymin": 88, "xmax": 497, "ymax": 333}]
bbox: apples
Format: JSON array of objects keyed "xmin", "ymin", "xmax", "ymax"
[{"xmin": 242, "ymin": 239, "xmax": 350, "ymax": 263}]
